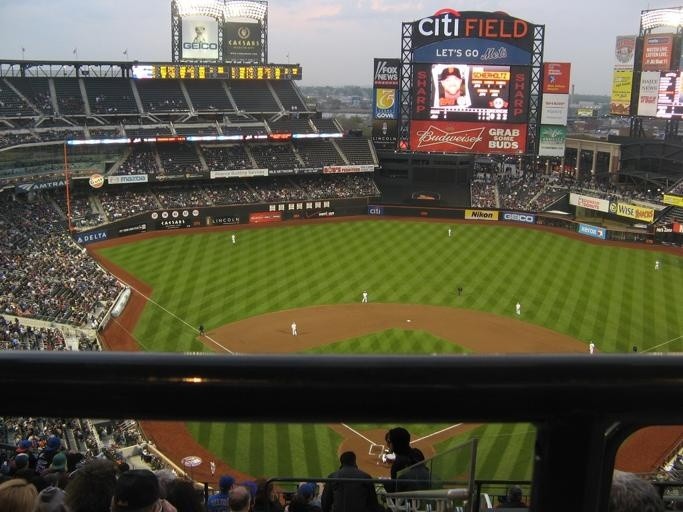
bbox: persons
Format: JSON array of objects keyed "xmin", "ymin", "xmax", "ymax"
[
  {"xmin": 290, "ymin": 321, "xmax": 297, "ymax": 337},
  {"xmin": 457, "ymin": 287, "xmax": 463, "ymax": 296},
  {"xmin": 588, "ymin": 341, "xmax": 595, "ymax": 354},
  {"xmin": 361, "ymin": 290, "xmax": 367, "ymax": 304},
  {"xmin": 197, "ymin": 325, "xmax": 205, "ymax": 336},
  {"xmin": 632, "ymin": 344, "xmax": 637, "ymax": 353},
  {"xmin": 0, "ymin": 227, "xmax": 136, "ymax": 511},
  {"xmin": 231, "ymin": 233, "xmax": 235, "ymax": 244},
  {"xmin": 654, "ymin": 260, "xmax": 659, "ymax": 270},
  {"xmin": 447, "ymin": 228, "xmax": 451, "ymax": 237},
  {"xmin": 135, "ymin": 427, "xmax": 683, "ymax": 512},
  {"xmin": 515, "ymin": 301, "xmax": 521, "ymax": 316},
  {"xmin": 0, "ymin": 146, "xmax": 683, "ymax": 228},
  {"xmin": 438, "ymin": 67, "xmax": 463, "ymax": 106},
  {"xmin": 0, "ymin": 92, "xmax": 220, "ymax": 146}
]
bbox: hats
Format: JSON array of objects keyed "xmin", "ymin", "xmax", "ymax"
[
  {"xmin": 389, "ymin": 427, "xmax": 411, "ymax": 457},
  {"xmin": 33, "ymin": 487, "xmax": 71, "ymax": 511},
  {"xmin": 53, "ymin": 453, "xmax": 68, "ymax": 471},
  {"xmin": 340, "ymin": 451, "xmax": 356, "ymax": 465},
  {"xmin": 44, "ymin": 437, "xmax": 60, "ymax": 451},
  {"xmin": 16, "ymin": 439, "xmax": 32, "ymax": 452},
  {"xmin": 440, "ymin": 67, "xmax": 462, "ymax": 81},
  {"xmin": 299, "ymin": 482, "xmax": 316, "ymax": 499},
  {"xmin": 219, "ymin": 476, "xmax": 235, "ymax": 486},
  {"xmin": 16, "ymin": 453, "xmax": 29, "ymax": 464}
]
